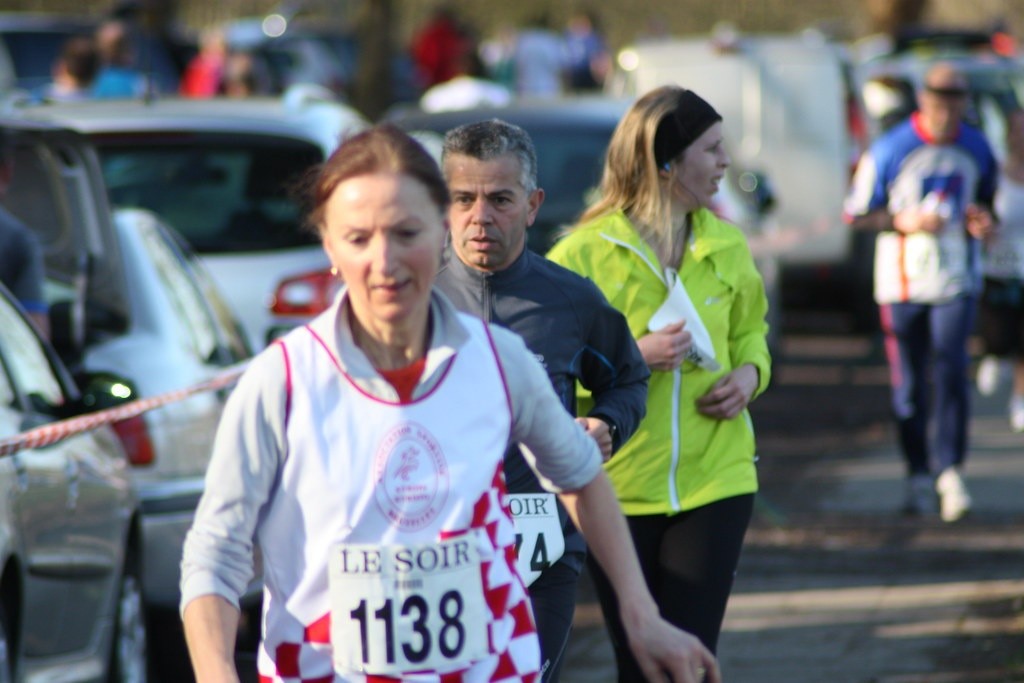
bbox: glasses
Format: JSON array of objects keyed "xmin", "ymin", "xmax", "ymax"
[{"xmin": 925, "ymin": 83, "xmax": 968, "ymax": 99}]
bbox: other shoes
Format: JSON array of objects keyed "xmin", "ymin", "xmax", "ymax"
[
  {"xmin": 908, "ymin": 475, "xmax": 937, "ymax": 513},
  {"xmin": 934, "ymin": 470, "xmax": 974, "ymax": 522}
]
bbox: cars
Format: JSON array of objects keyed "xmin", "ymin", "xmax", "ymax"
[{"xmin": 2, "ymin": 5, "xmax": 1023, "ymax": 682}]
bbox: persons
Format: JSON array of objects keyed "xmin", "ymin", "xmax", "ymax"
[
  {"xmin": 545, "ymin": 86, "xmax": 772, "ymax": 683},
  {"xmin": 974, "ymin": 107, "xmax": 1024, "ymax": 430},
  {"xmin": 431, "ymin": 120, "xmax": 654, "ymax": 683},
  {"xmin": 177, "ymin": 125, "xmax": 720, "ymax": 683},
  {"xmin": 14, "ymin": 5, "xmax": 615, "ymax": 111},
  {"xmin": 842, "ymin": 66, "xmax": 1000, "ymax": 523},
  {"xmin": 0, "ymin": 206, "xmax": 47, "ymax": 344}
]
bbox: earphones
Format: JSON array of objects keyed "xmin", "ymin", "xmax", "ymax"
[{"xmin": 663, "ymin": 163, "xmax": 671, "ymax": 172}]
